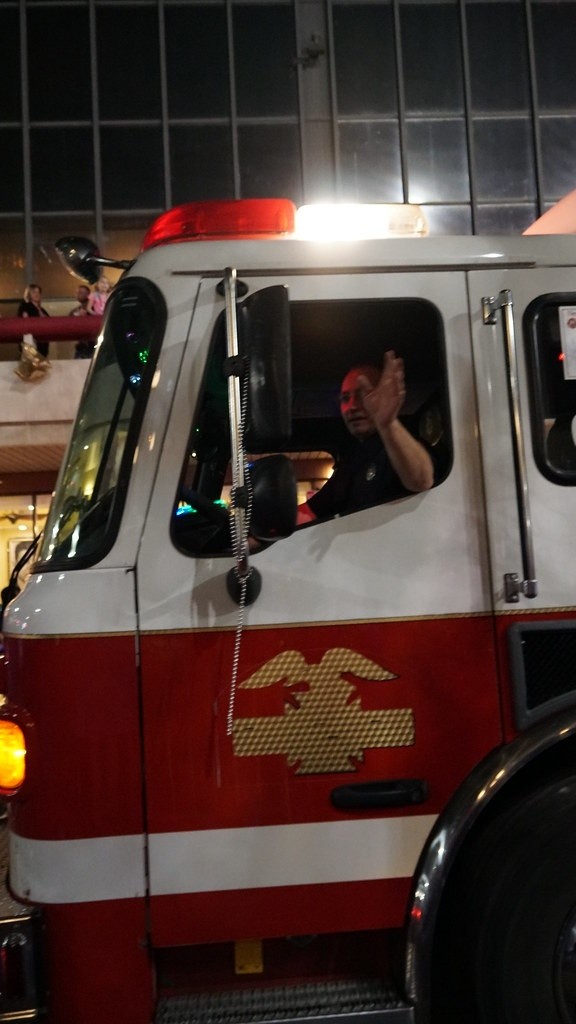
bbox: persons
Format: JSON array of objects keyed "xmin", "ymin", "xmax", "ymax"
[
  {"xmin": 68, "ymin": 286, "xmax": 97, "ymax": 359},
  {"xmin": 87, "ymin": 276, "xmax": 110, "ymax": 315},
  {"xmin": 249, "ymin": 350, "xmax": 435, "ymax": 550},
  {"xmin": 18, "ymin": 284, "xmax": 51, "ymax": 359}
]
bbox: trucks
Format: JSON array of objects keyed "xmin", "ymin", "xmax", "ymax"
[{"xmin": 1, "ymin": 200, "xmax": 576, "ymax": 1022}]
